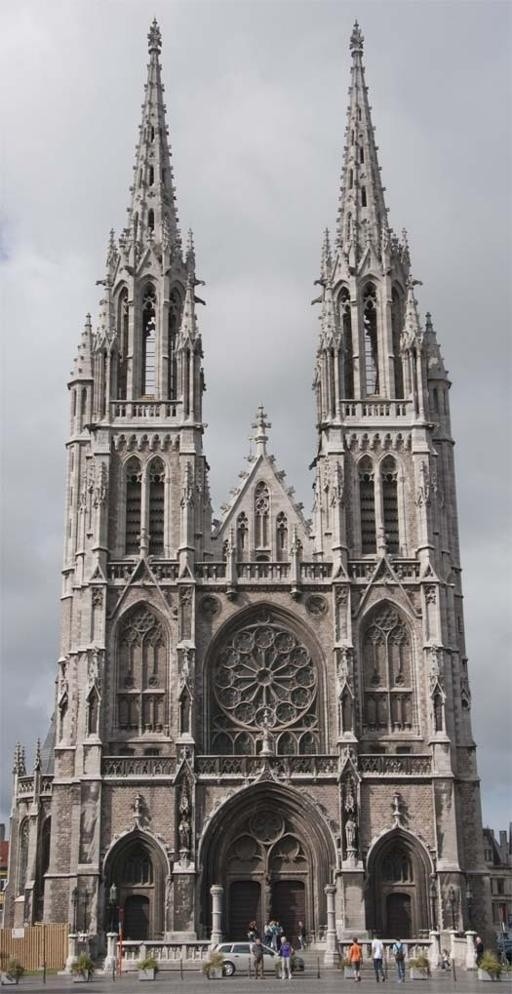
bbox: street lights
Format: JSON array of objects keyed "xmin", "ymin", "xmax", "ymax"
[
  {"xmin": 109, "ymin": 882, "xmax": 120, "ymax": 932},
  {"xmin": 465, "ymin": 880, "xmax": 473, "ymax": 929}
]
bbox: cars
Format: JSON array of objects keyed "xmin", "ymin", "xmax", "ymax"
[{"xmin": 211, "ymin": 942, "xmax": 304, "ymax": 976}]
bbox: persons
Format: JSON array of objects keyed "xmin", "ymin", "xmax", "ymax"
[
  {"xmin": 371, "ymin": 935, "xmax": 385, "ymax": 982},
  {"xmin": 476, "ymin": 936, "xmax": 484, "ymax": 968},
  {"xmin": 441, "ymin": 949, "xmax": 451, "ymax": 969},
  {"xmin": 264, "ymin": 920, "xmax": 283, "ymax": 951},
  {"xmin": 348, "ymin": 937, "xmax": 362, "ymax": 982},
  {"xmin": 248, "ymin": 921, "xmax": 257, "ymax": 942},
  {"xmin": 279, "ymin": 936, "xmax": 294, "ymax": 980},
  {"xmin": 252, "ymin": 938, "xmax": 266, "ymax": 979},
  {"xmin": 393, "ymin": 937, "xmax": 406, "ymax": 983},
  {"xmin": 298, "ymin": 921, "xmax": 308, "ymax": 951}
]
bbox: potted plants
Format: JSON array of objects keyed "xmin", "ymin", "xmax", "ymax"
[
  {"xmin": 477, "ymin": 949, "xmax": 503, "ymax": 981},
  {"xmin": 69, "ymin": 953, "xmax": 98, "ymax": 983},
  {"xmin": 406, "ymin": 956, "xmax": 430, "ymax": 979},
  {"xmin": 135, "ymin": 958, "xmax": 160, "ymax": 980},
  {"xmin": 199, "ymin": 952, "xmax": 226, "ymax": 978},
  {"xmin": 339, "ymin": 955, "xmax": 363, "ymax": 979},
  {"xmin": 1, "ymin": 957, "xmax": 26, "ymax": 985}
]
